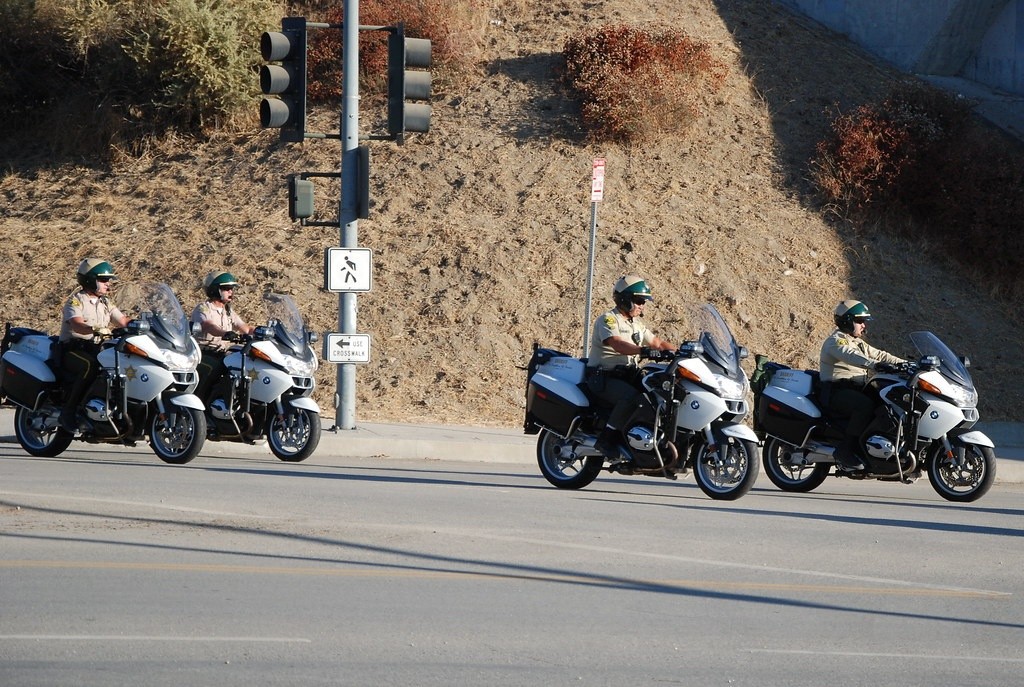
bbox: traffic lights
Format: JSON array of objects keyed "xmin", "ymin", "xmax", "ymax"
[
  {"xmin": 260, "ymin": 16, "xmax": 307, "ymax": 143},
  {"xmin": 288, "ymin": 176, "xmax": 314, "ymax": 223},
  {"xmin": 387, "ymin": 21, "xmax": 432, "ymax": 146}
]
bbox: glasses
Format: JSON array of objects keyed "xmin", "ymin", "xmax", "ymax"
[
  {"xmin": 97, "ymin": 277, "xmax": 109, "ymax": 282},
  {"xmin": 633, "ymin": 298, "xmax": 645, "ymax": 305},
  {"xmin": 853, "ymin": 318, "xmax": 865, "ymax": 324},
  {"xmin": 221, "ymin": 286, "xmax": 233, "ymax": 291}
]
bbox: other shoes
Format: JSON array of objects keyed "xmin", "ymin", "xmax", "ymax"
[
  {"xmin": 594, "ymin": 437, "xmax": 621, "ymax": 458},
  {"xmin": 833, "ymin": 451, "xmax": 864, "ymax": 469}
]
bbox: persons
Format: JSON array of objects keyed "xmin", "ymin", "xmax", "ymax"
[
  {"xmin": 59, "ymin": 257, "xmax": 130, "ymax": 435},
  {"xmin": 191, "ymin": 270, "xmax": 257, "ymax": 436},
  {"xmin": 583, "ymin": 275, "xmax": 679, "ymax": 463},
  {"xmin": 820, "ymin": 300, "xmax": 909, "ymax": 472}
]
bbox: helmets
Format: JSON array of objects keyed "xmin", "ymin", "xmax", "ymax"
[
  {"xmin": 613, "ymin": 275, "xmax": 652, "ymax": 312},
  {"xmin": 834, "ymin": 300, "xmax": 870, "ymax": 332},
  {"xmin": 203, "ymin": 271, "xmax": 239, "ymax": 300},
  {"xmin": 76, "ymin": 258, "xmax": 118, "ymax": 292}
]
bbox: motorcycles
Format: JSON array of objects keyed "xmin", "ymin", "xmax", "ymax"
[
  {"xmin": 0, "ymin": 284, "xmax": 208, "ymax": 464},
  {"xmin": 756, "ymin": 331, "xmax": 996, "ymax": 502},
  {"xmin": 522, "ymin": 302, "xmax": 760, "ymax": 501},
  {"xmin": 189, "ymin": 293, "xmax": 322, "ymax": 462}
]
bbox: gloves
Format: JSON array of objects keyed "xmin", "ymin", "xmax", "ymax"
[
  {"xmin": 641, "ymin": 346, "xmax": 665, "ymax": 361},
  {"xmin": 222, "ymin": 331, "xmax": 239, "ymax": 345},
  {"xmin": 92, "ymin": 325, "xmax": 111, "ymax": 338},
  {"xmin": 874, "ymin": 362, "xmax": 898, "ymax": 375}
]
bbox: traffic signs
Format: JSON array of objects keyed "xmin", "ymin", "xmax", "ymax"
[{"xmin": 321, "ymin": 331, "xmax": 371, "ymax": 364}]
